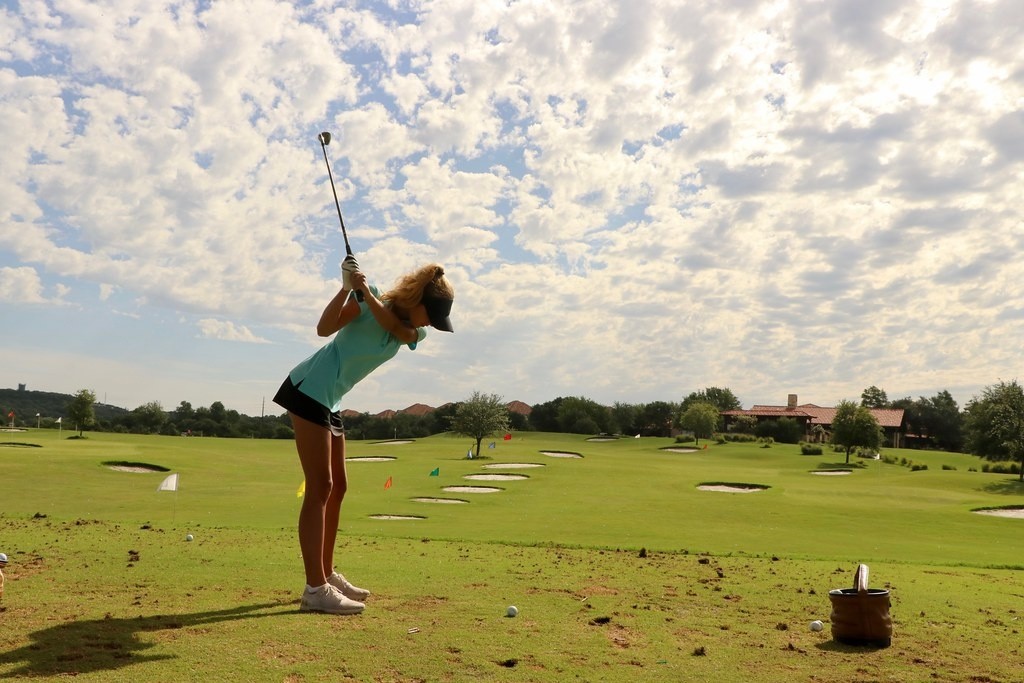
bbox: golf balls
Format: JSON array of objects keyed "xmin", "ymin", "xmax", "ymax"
[
  {"xmin": 507, "ymin": 606, "xmax": 518, "ymax": 616},
  {"xmin": 187, "ymin": 534, "xmax": 193, "ymax": 541},
  {"xmin": 809, "ymin": 620, "xmax": 824, "ymax": 631}
]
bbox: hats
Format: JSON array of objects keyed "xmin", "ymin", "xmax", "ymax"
[{"xmin": 419, "ymin": 295, "xmax": 454, "ymax": 334}]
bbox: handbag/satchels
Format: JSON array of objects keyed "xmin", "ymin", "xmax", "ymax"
[{"xmin": 827, "ymin": 564, "xmax": 892, "ymax": 648}]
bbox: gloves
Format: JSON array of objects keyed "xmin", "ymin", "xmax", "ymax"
[{"xmin": 341, "ymin": 254, "xmax": 359, "ymax": 290}]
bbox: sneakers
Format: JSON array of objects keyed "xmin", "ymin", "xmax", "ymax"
[
  {"xmin": 300, "ymin": 584, "xmax": 365, "ymax": 613},
  {"xmin": 329, "ymin": 574, "xmax": 371, "ymax": 600}
]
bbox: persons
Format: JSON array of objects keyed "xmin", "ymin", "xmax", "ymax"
[{"xmin": 273, "ymin": 255, "xmax": 455, "ymax": 614}]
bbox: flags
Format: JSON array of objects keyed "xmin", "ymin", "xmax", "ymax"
[
  {"xmin": 489, "ymin": 443, "xmax": 494, "ymax": 448},
  {"xmin": 384, "ymin": 477, "xmax": 391, "ymax": 490},
  {"xmin": 297, "ymin": 481, "xmax": 305, "ymax": 497},
  {"xmin": 430, "ymin": 468, "xmax": 438, "ymax": 477},
  {"xmin": 157, "ymin": 474, "xmax": 177, "ymax": 491}
]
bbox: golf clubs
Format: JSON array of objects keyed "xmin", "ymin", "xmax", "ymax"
[{"xmin": 317, "ymin": 130, "xmax": 365, "ymax": 303}]
